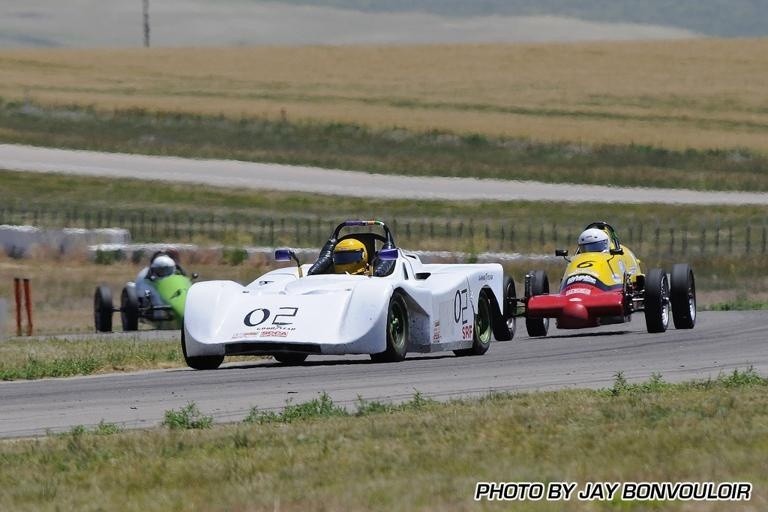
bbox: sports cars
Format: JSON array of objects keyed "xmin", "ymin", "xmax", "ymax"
[{"xmin": 181, "ymin": 219, "xmax": 504, "ymax": 371}]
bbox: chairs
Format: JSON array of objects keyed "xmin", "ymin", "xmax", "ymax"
[{"xmin": 332, "ymin": 234, "xmax": 392, "ymax": 275}]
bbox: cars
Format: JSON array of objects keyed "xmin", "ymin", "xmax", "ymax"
[{"xmin": 493, "ymin": 221, "xmax": 696, "ymax": 342}]
